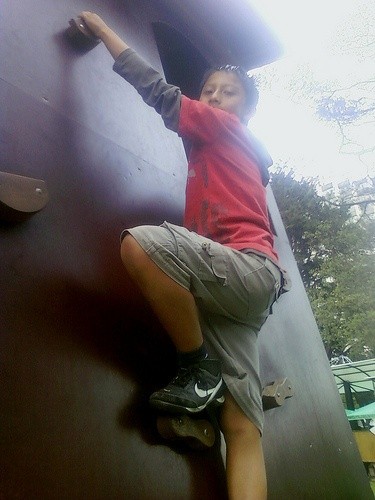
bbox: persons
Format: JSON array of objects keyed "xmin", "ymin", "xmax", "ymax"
[{"xmin": 78, "ymin": 9, "xmax": 291, "ymax": 500}]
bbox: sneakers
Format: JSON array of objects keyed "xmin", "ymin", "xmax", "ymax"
[{"xmin": 147, "ymin": 366, "xmax": 228, "ymax": 412}]
